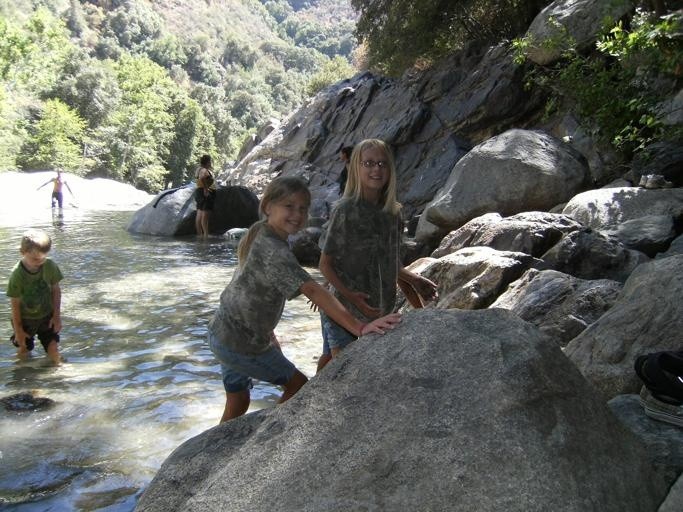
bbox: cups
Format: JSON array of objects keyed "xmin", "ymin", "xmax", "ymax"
[{"xmin": 361, "ymin": 159, "xmax": 388, "ymax": 169}]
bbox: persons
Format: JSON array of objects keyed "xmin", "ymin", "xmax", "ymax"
[
  {"xmin": 323, "ymin": 138, "xmax": 438, "ymax": 357},
  {"xmin": 306, "ymin": 256, "xmax": 424, "ymax": 373},
  {"xmin": 6, "ymin": 228, "xmax": 64, "ymax": 365},
  {"xmin": 337, "ymin": 146, "xmax": 354, "ymax": 195},
  {"xmin": 195, "ymin": 154, "xmax": 216, "ymax": 239},
  {"xmin": 36, "ymin": 173, "xmax": 73, "ymax": 209},
  {"xmin": 207, "ymin": 176, "xmax": 403, "ymax": 424}
]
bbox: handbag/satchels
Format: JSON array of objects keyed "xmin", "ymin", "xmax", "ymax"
[{"xmin": 359, "ymin": 322, "xmax": 367, "ymax": 336}]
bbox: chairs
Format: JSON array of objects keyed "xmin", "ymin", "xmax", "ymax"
[
  {"xmin": 639, "ymin": 385, "xmax": 651, "ymax": 408},
  {"xmin": 643, "ymin": 394, "xmax": 683, "ymax": 426}
]
